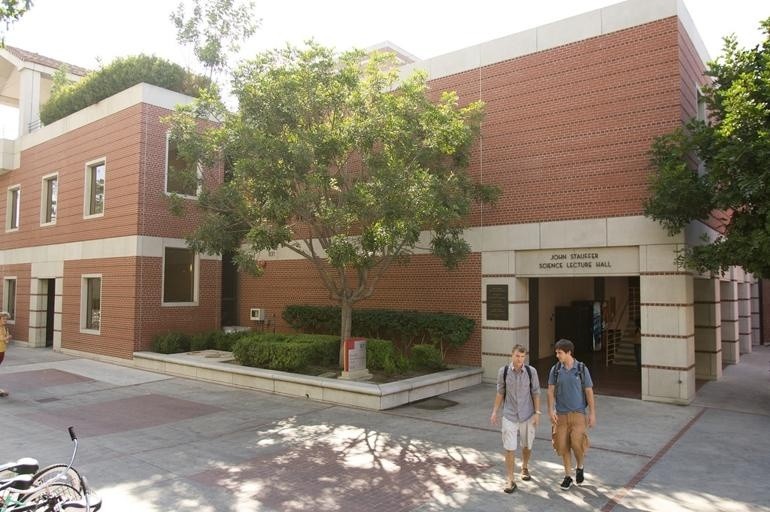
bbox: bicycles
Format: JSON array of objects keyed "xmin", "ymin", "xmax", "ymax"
[{"xmin": 0, "ymin": 426, "xmax": 101, "ymax": 512}]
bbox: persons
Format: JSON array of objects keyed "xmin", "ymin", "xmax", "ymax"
[
  {"xmin": 546, "ymin": 339, "xmax": 597, "ymax": 490},
  {"xmin": 0, "ymin": 312, "xmax": 13, "ymax": 397},
  {"xmin": 490, "ymin": 344, "xmax": 542, "ymax": 493},
  {"xmin": 633, "ymin": 320, "xmax": 641, "ymax": 373}
]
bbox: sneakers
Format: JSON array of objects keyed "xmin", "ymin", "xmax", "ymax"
[
  {"xmin": 521, "ymin": 468, "xmax": 530, "ymax": 480},
  {"xmin": 574, "ymin": 465, "xmax": 584, "ymax": 484},
  {"xmin": 561, "ymin": 476, "xmax": 573, "ymax": 491},
  {"xmin": 504, "ymin": 482, "xmax": 516, "ymax": 493}
]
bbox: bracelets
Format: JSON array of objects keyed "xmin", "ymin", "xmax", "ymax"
[{"xmin": 535, "ymin": 410, "xmax": 541, "ymax": 414}]
